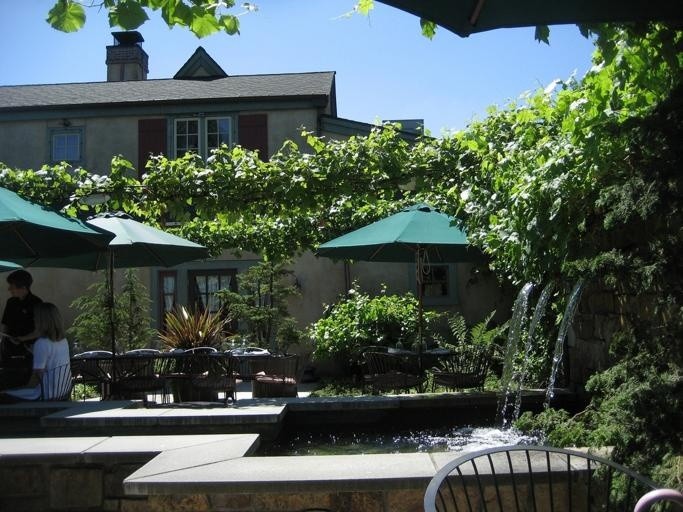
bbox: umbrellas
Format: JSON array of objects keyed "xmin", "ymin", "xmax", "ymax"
[
  {"xmin": 8, "ymin": 210, "xmax": 212, "ymax": 401},
  {"xmin": 314, "ymin": 203, "xmax": 477, "ymax": 393},
  {"xmin": 0, "ymin": 187, "xmax": 117, "ymax": 259}
]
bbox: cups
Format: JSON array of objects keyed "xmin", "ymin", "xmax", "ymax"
[{"xmin": 391, "ymin": 336, "xmax": 429, "ymax": 354}]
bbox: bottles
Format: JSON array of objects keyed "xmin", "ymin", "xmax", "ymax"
[{"xmin": 229, "ymin": 338, "xmax": 248, "ymax": 355}]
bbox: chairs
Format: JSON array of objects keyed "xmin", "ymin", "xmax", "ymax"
[
  {"xmin": 362, "ymin": 353, "xmax": 426, "ymax": 392},
  {"xmin": 0, "ymin": 343, "xmax": 300, "ymax": 402},
  {"xmin": 433, "ymin": 347, "xmax": 496, "ymax": 392},
  {"xmin": 423, "ymin": 445, "xmax": 683, "ymax": 512},
  {"xmin": 358, "ymin": 346, "xmax": 401, "ymax": 390}
]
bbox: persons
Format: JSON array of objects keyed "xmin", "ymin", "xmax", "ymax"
[
  {"xmin": 0, "ymin": 302, "xmax": 72, "ymax": 400},
  {"xmin": 0, "ymin": 270, "xmax": 43, "ymax": 403}
]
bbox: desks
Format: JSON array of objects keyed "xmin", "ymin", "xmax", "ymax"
[{"xmin": 399, "ymin": 349, "xmax": 450, "ymax": 389}]
